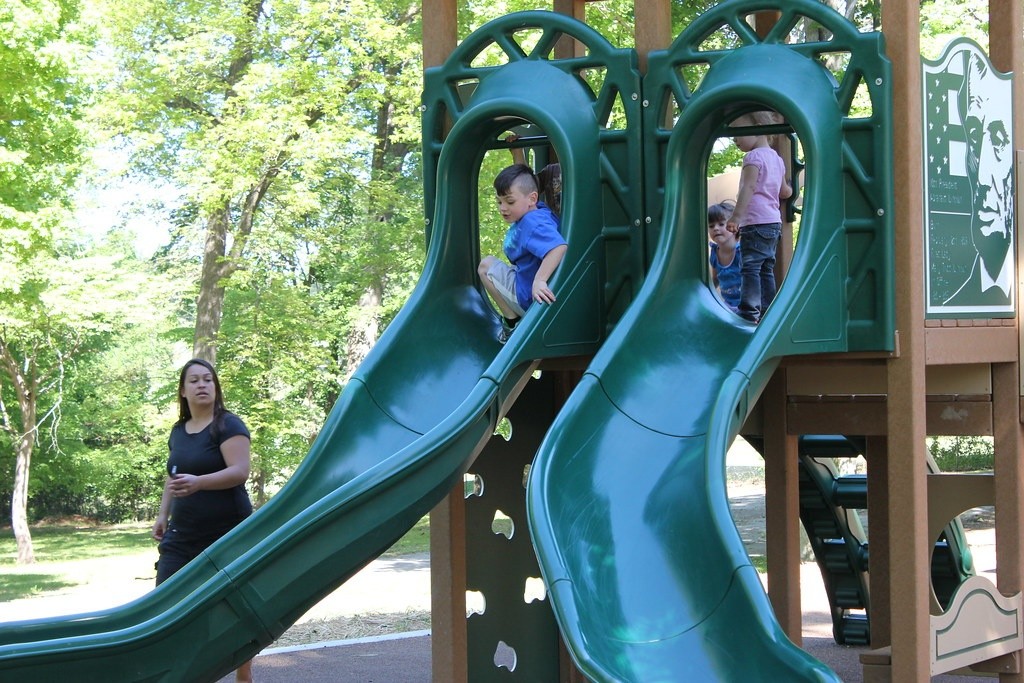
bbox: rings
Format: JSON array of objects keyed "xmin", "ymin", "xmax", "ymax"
[{"xmin": 185, "ymin": 488, "xmax": 190, "ymax": 493}]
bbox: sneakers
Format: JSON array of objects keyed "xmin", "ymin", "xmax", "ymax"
[{"xmin": 496, "ymin": 316, "xmax": 519, "ymax": 344}]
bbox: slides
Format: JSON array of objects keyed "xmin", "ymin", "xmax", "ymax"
[
  {"xmin": 523, "ymin": 282, "xmax": 847, "ymax": 683},
  {"xmin": 0, "ymin": 279, "xmax": 602, "ymax": 683}
]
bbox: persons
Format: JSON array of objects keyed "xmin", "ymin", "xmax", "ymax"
[
  {"xmin": 476, "ymin": 164, "xmax": 568, "ymax": 345},
  {"xmin": 151, "ymin": 358, "xmax": 255, "ymax": 683},
  {"xmin": 725, "ymin": 109, "xmax": 793, "ymax": 324},
  {"xmin": 708, "ymin": 200, "xmax": 743, "ymax": 314},
  {"xmin": 506, "ymin": 134, "xmax": 561, "ymax": 220}
]
